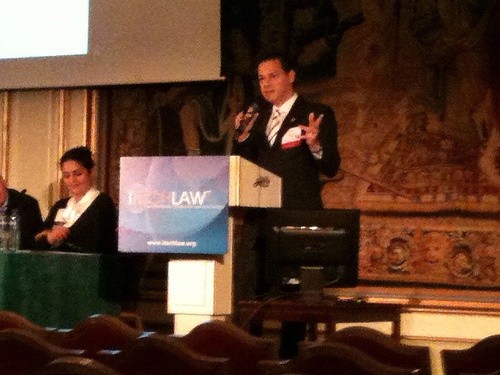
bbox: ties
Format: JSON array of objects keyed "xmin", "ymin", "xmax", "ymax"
[{"xmin": 267, "ymin": 109, "xmax": 282, "ymax": 147}]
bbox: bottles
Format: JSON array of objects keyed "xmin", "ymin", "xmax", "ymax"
[{"xmin": 0, "ymin": 207, "xmax": 21, "ymax": 252}]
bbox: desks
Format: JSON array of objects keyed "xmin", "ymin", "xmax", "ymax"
[
  {"xmin": 237, "ymin": 298, "xmax": 404, "ymax": 341},
  {"xmin": 0, "ymin": 247, "xmax": 119, "ymax": 326}
]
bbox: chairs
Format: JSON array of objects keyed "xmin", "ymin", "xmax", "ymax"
[
  {"xmin": 439, "ymin": 332, "xmax": 500, "ymax": 375},
  {"xmin": 0, "ymin": 308, "xmax": 433, "ymax": 375}
]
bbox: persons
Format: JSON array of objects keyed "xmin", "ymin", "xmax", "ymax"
[
  {"xmin": 232, "ymin": 51, "xmax": 341, "ymax": 359},
  {"xmin": 30, "ymin": 145, "xmax": 117, "ymax": 257},
  {"xmin": 0, "ymin": 180, "xmax": 42, "ymax": 250}
]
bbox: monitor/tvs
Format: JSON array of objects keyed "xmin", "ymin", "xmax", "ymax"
[{"xmin": 257, "ymin": 208, "xmax": 358, "ymax": 306}]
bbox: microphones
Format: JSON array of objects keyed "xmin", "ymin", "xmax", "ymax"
[{"xmin": 234, "ymin": 102, "xmax": 259, "ymax": 140}]
